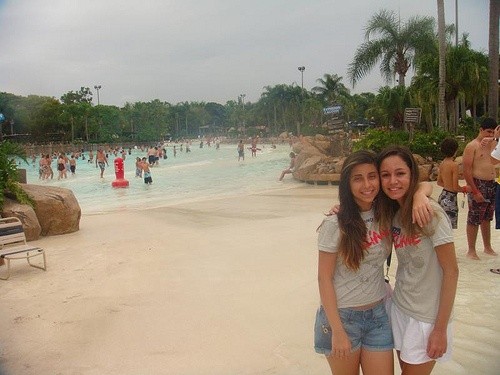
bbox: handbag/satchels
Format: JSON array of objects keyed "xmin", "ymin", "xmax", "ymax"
[{"xmin": 383, "ymin": 275, "xmax": 394, "ymax": 317}]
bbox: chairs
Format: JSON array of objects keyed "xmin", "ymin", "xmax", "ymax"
[{"xmin": 0, "ymin": 217, "xmax": 46, "ymax": 280}]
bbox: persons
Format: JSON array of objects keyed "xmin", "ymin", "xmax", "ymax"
[
  {"xmin": 27, "ymin": 136, "xmax": 298, "ymax": 184},
  {"xmin": 436, "ymin": 138, "xmax": 472, "ymax": 229},
  {"xmin": 330, "ymin": 144, "xmax": 459, "ymax": 375},
  {"xmin": 463, "ymin": 118, "xmax": 499, "ymax": 261},
  {"xmin": 490, "ymin": 124, "xmax": 500, "ymax": 229},
  {"xmin": 313, "ymin": 149, "xmax": 433, "ymax": 375}
]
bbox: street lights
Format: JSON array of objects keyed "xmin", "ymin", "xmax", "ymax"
[
  {"xmin": 94, "ymin": 85, "xmax": 102, "ymax": 135},
  {"xmin": 298, "ymin": 66, "xmax": 305, "ymax": 123}
]
